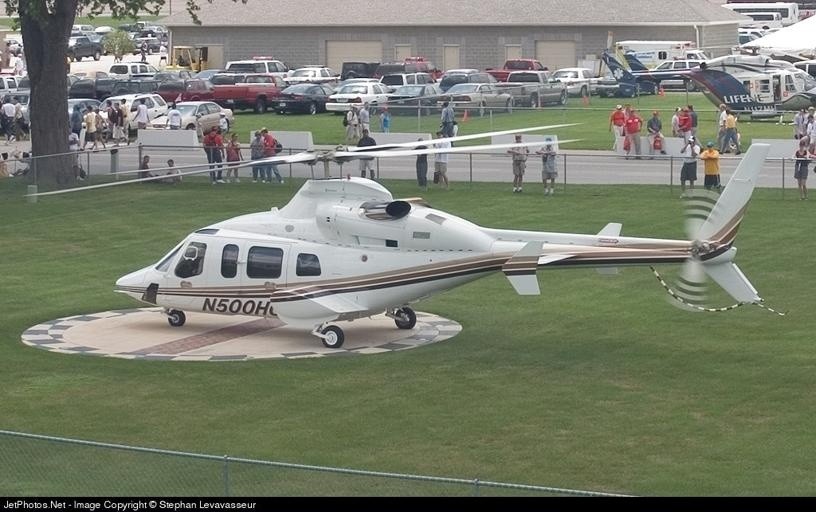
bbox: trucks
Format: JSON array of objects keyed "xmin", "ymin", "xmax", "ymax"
[{"xmin": 613, "ymin": 40, "xmax": 708, "ymax": 70}]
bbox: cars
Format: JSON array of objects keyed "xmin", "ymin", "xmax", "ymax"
[{"xmin": 0, "ymin": 23, "xmax": 237, "ymax": 142}]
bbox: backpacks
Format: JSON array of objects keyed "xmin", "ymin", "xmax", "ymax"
[
  {"xmin": 342, "ymin": 110, "xmax": 355, "ymax": 127},
  {"xmin": 205, "ymin": 132, "xmax": 221, "ymax": 155}
]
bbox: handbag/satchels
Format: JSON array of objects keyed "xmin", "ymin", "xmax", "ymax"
[
  {"xmin": 623, "ymin": 136, "xmax": 631, "ymax": 150},
  {"xmin": 276, "ymin": 143, "xmax": 282, "ymax": 154},
  {"xmin": 209, "ymin": 177, "xmax": 286, "ymax": 185},
  {"xmin": 654, "ymin": 134, "xmax": 662, "ymax": 150}
]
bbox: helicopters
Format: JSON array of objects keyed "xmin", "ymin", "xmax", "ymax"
[
  {"xmin": 599, "ymin": 46, "xmax": 816, "ymax": 127},
  {"xmin": 25, "ymin": 122, "xmax": 778, "ymax": 351}
]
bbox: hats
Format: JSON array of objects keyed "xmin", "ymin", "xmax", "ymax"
[
  {"xmin": 707, "ymin": 141, "xmax": 714, "ymax": 148},
  {"xmin": 688, "ymin": 135, "xmax": 697, "ymax": 143},
  {"xmin": 653, "ymin": 110, "xmax": 659, "ymax": 116},
  {"xmin": 683, "ymin": 107, "xmax": 690, "ymax": 112},
  {"xmin": 232, "ymin": 133, "xmax": 239, "ymax": 138},
  {"xmin": 220, "ymin": 113, "xmax": 226, "ymax": 116},
  {"xmin": 675, "ymin": 106, "xmax": 682, "ymax": 112},
  {"xmin": 630, "ymin": 108, "xmax": 635, "ymax": 112},
  {"xmin": 211, "ymin": 125, "xmax": 220, "ymax": 131},
  {"xmin": 616, "ymin": 104, "xmax": 623, "ymax": 111},
  {"xmin": 255, "ymin": 130, "xmax": 261, "ymax": 136},
  {"xmin": 260, "ymin": 127, "xmax": 269, "ymax": 133}
]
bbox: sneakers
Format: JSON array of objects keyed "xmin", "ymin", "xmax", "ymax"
[{"xmin": 512, "ymin": 186, "xmax": 555, "ymax": 196}]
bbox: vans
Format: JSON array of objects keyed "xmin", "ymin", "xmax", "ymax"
[{"xmin": 719, "ymin": 1, "xmax": 816, "ymax": 47}]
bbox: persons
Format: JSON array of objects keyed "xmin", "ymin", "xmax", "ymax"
[
  {"xmin": 0, "ymin": 40, "xmax": 284, "ymax": 185},
  {"xmin": 792, "ymin": 107, "xmax": 816, "ymax": 200},
  {"xmin": 535, "ymin": 138, "xmax": 558, "ymax": 195},
  {"xmin": 507, "ymin": 134, "xmax": 530, "ymax": 192},
  {"xmin": 413, "ymin": 102, "xmax": 455, "ymax": 191},
  {"xmin": 608, "ymin": 103, "xmax": 742, "ymax": 198},
  {"xmin": 345, "ymin": 104, "xmax": 390, "ymax": 179}
]
